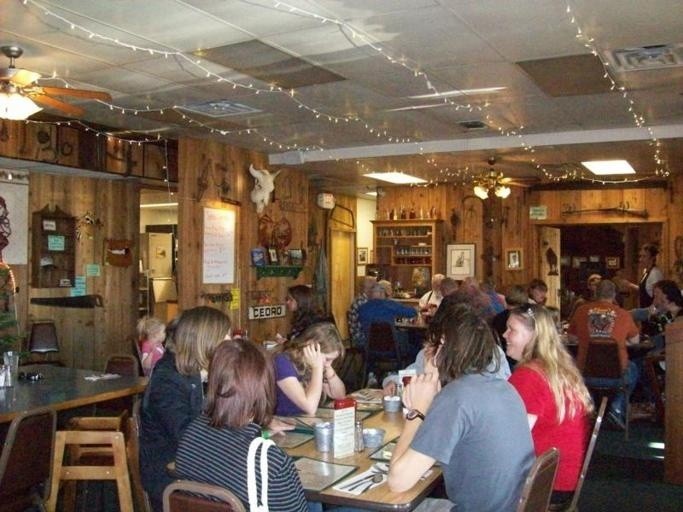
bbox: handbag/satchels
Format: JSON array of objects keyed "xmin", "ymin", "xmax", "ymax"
[{"xmin": 339, "ymin": 348, "xmax": 362, "ymax": 381}]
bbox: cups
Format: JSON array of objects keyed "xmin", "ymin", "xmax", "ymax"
[
  {"xmin": 334, "ymin": 397, "xmax": 356, "ymax": 410},
  {"xmin": 312, "ymin": 422, "xmax": 334, "ymax": 453},
  {"xmin": 361, "ymin": 427, "xmax": 386, "ymax": 448},
  {"xmin": 2, "ymin": 350, "xmax": 19, "ymax": 377},
  {"xmin": 382, "ymin": 395, "xmax": 401, "ymax": 412}
]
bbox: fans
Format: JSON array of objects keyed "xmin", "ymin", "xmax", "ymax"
[
  {"xmin": 1, "ymin": 42, "xmax": 109, "ymax": 123},
  {"xmin": 450, "ymin": 153, "xmax": 542, "ymax": 199}
]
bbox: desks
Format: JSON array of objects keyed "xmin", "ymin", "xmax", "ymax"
[{"xmin": 257, "ymin": 387, "xmax": 447, "ymax": 512}]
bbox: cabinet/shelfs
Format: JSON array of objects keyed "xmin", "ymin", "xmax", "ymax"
[{"xmin": 369, "ymin": 219, "xmax": 444, "ymax": 297}]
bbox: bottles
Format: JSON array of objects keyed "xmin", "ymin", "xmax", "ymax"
[
  {"xmin": 375, "ymin": 205, "xmax": 433, "ymax": 266},
  {"xmin": 0, "ymin": 364, "xmax": 13, "ymax": 388},
  {"xmin": 353, "ymin": 419, "xmax": 364, "ymax": 452}
]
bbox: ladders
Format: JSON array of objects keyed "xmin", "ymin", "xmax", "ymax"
[{"xmin": 137, "ymin": 270, "xmax": 149, "ymax": 319}]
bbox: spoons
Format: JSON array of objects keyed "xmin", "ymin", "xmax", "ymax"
[{"xmin": 361, "ymin": 473, "xmax": 383, "ymax": 495}]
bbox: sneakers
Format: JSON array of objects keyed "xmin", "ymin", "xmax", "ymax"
[{"xmin": 606, "ymin": 409, "xmax": 626, "ymax": 429}]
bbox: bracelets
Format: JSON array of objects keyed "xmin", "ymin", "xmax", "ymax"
[{"xmin": 325, "ymin": 372, "xmax": 336, "ymax": 381}]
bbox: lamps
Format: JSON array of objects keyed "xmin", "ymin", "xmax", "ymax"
[
  {"xmin": 0, "ymin": 90, "xmax": 43, "ymax": 123},
  {"xmin": 470, "ymin": 178, "xmax": 510, "ymax": 199}
]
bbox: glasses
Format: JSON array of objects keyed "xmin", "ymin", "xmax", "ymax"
[{"xmin": 521, "ymin": 304, "xmax": 536, "ymax": 322}]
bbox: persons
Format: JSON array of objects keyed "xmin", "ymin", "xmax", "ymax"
[
  {"xmin": 139, "ymin": 307, "xmax": 231, "ymax": 512},
  {"xmin": 175, "ymin": 339, "xmax": 310, "ymax": 512},
  {"xmin": 329, "ymin": 305, "xmax": 537, "ymax": 512},
  {"xmin": 503, "ymin": 303, "xmax": 593, "ymax": 504},
  {"xmin": 271, "ymin": 321, "xmax": 346, "ymax": 415},
  {"xmin": 350, "ymin": 246, "xmax": 682, "ymax": 430},
  {"xmin": 140, "ymin": 286, "xmax": 334, "ymax": 376}
]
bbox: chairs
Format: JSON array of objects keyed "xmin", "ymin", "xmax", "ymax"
[
  {"xmin": 93, "ymin": 354, "xmax": 135, "ymax": 422},
  {"xmin": 514, "ymin": 444, "xmax": 559, "ymax": 512},
  {"xmin": 25, "ymin": 319, "xmax": 64, "ymax": 366},
  {"xmin": 581, "ymin": 336, "xmax": 632, "ymax": 440},
  {"xmin": 548, "ymin": 396, "xmax": 608, "ymax": 512},
  {"xmin": 338, "ymin": 310, "xmax": 365, "ymax": 381},
  {"xmin": 364, "ymin": 320, "xmax": 414, "ymax": 387},
  {"xmin": 0, "ymin": 406, "xmax": 59, "ymax": 511},
  {"xmin": 159, "ymin": 478, "xmax": 244, "ymax": 510}
]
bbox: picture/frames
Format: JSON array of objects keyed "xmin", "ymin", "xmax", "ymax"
[
  {"xmin": 604, "ymin": 256, "xmax": 619, "ymax": 269},
  {"xmin": 356, "ymin": 248, "xmax": 368, "ymax": 266},
  {"xmin": 505, "ymin": 247, "xmax": 524, "ymax": 271},
  {"xmin": 266, "ymin": 246, "xmax": 278, "ymax": 264},
  {"xmin": 445, "ymin": 243, "xmax": 475, "ymax": 283}
]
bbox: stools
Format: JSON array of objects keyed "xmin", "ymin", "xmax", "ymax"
[{"xmin": 44, "ymin": 413, "xmax": 135, "ymax": 510}]
